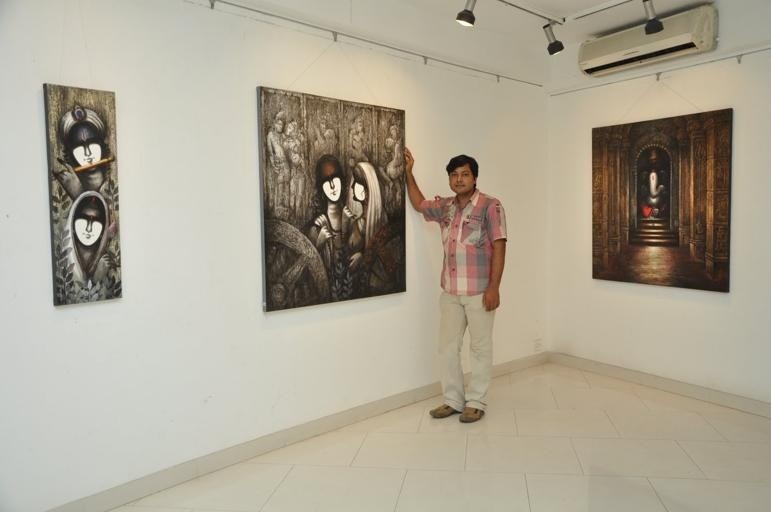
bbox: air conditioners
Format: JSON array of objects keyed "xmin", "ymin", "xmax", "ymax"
[{"xmin": 577, "ymin": 5, "xmax": 719, "ymax": 79}]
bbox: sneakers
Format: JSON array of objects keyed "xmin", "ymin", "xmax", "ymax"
[
  {"xmin": 429, "ymin": 404, "xmax": 460, "ymax": 419},
  {"xmin": 459, "ymin": 407, "xmax": 484, "ymax": 422}
]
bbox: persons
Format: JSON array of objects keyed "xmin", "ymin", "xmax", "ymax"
[
  {"xmin": 62, "ymin": 189, "xmax": 112, "ymax": 288},
  {"xmin": 403, "ymin": 145, "xmax": 506, "ymax": 422},
  {"xmin": 56, "ymin": 104, "xmax": 116, "ymax": 199},
  {"xmin": 268, "ymin": 117, "xmax": 402, "ymax": 304}
]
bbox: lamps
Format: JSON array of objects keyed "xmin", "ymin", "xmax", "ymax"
[
  {"xmin": 454, "ymin": 0, "xmax": 482, "ymax": 27},
  {"xmin": 642, "ymin": 1, "xmax": 666, "ymax": 36},
  {"xmin": 543, "ymin": 18, "xmax": 565, "ymax": 57}
]
